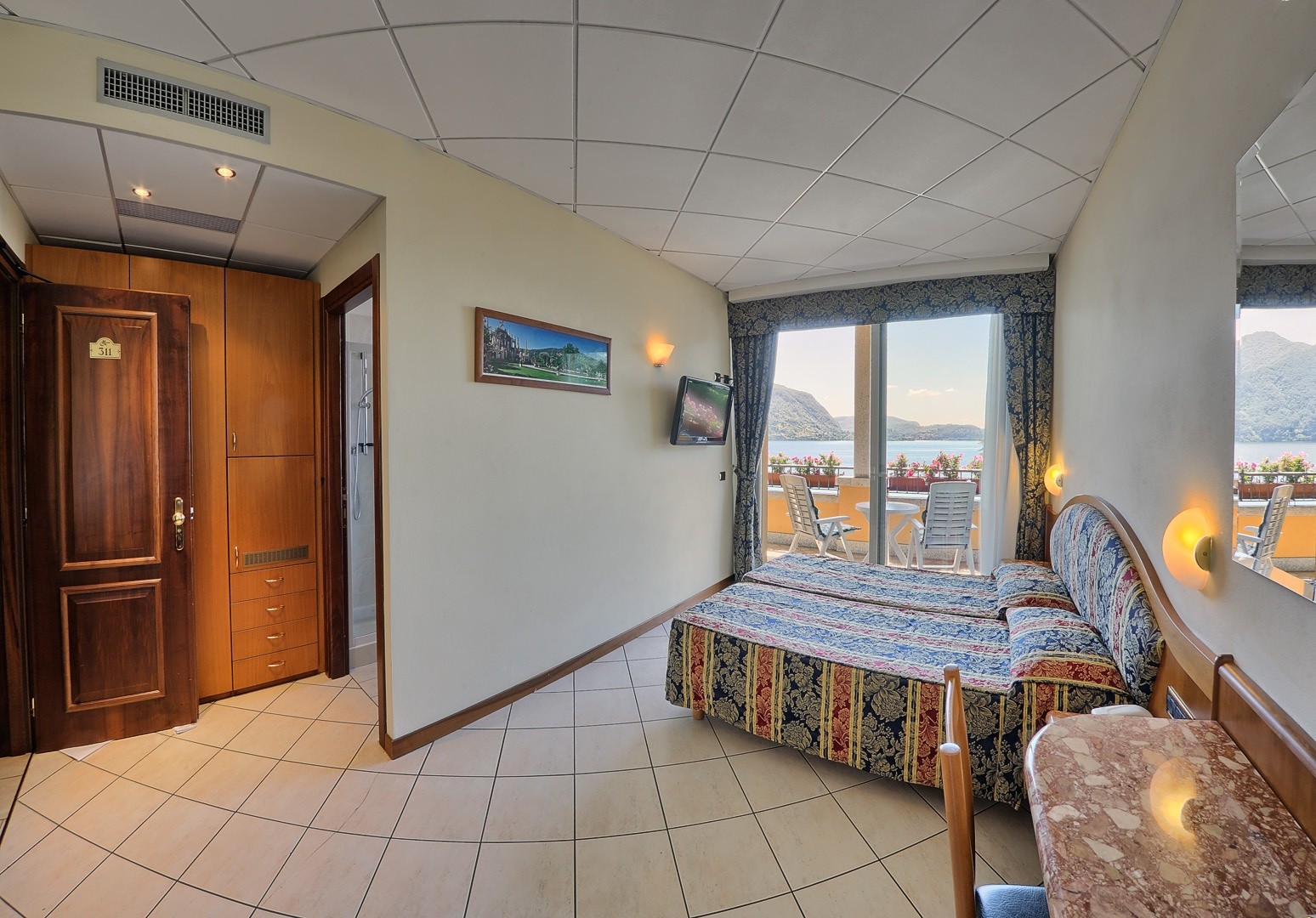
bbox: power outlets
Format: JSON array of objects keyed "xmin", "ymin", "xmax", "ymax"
[{"xmin": 1166, "ymin": 686, "xmax": 1194, "ymax": 720}]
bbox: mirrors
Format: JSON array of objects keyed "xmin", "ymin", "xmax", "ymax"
[{"xmin": 1230, "ymin": 68, "xmax": 1316, "ymax": 605}]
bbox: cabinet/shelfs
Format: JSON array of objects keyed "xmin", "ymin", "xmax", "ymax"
[{"xmin": 23, "ymin": 244, "xmax": 328, "ymax": 706}]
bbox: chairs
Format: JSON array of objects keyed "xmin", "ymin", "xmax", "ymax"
[
  {"xmin": 779, "ymin": 474, "xmax": 861, "ymax": 560},
  {"xmin": 907, "ymin": 481, "xmax": 976, "ymax": 575},
  {"xmin": 1237, "ymin": 485, "xmax": 1293, "ymax": 566},
  {"xmin": 939, "ymin": 663, "xmax": 1049, "ymax": 918}
]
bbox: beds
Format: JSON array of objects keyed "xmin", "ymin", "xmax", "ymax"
[{"xmin": 664, "ymin": 493, "xmax": 1234, "ymax": 810}]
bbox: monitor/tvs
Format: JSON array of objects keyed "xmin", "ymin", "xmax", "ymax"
[{"xmin": 669, "ymin": 375, "xmax": 734, "ymax": 446}]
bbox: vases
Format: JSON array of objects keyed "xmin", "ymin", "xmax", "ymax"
[
  {"xmin": 927, "ymin": 476, "xmax": 980, "ymax": 495},
  {"xmin": 767, "ymin": 473, "xmax": 837, "ymax": 488},
  {"xmin": 1238, "ymin": 481, "xmax": 1316, "ymax": 500},
  {"xmin": 888, "ymin": 476, "xmax": 926, "ymax": 491}
]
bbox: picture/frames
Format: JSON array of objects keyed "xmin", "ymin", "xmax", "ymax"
[{"xmin": 475, "ymin": 306, "xmax": 613, "ymax": 396}]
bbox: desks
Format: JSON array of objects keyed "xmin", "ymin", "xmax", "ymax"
[
  {"xmin": 1022, "ymin": 716, "xmax": 1316, "ymax": 918},
  {"xmin": 855, "ymin": 501, "xmax": 920, "ymax": 566}
]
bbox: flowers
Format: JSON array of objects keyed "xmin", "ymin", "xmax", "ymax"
[
  {"xmin": 766, "ymin": 451, "xmax": 842, "ymax": 475},
  {"xmin": 888, "ymin": 453, "xmax": 983, "ymax": 478},
  {"xmin": 1236, "ymin": 451, "xmax": 1316, "ymax": 485}
]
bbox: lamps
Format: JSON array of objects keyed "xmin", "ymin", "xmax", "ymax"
[
  {"xmin": 1043, "ymin": 465, "xmax": 1064, "ymax": 496},
  {"xmin": 1149, "ymin": 761, "xmax": 1196, "ymax": 844},
  {"xmin": 1162, "ymin": 507, "xmax": 1211, "ymax": 591},
  {"xmin": 650, "ymin": 344, "xmax": 674, "ymax": 367}
]
